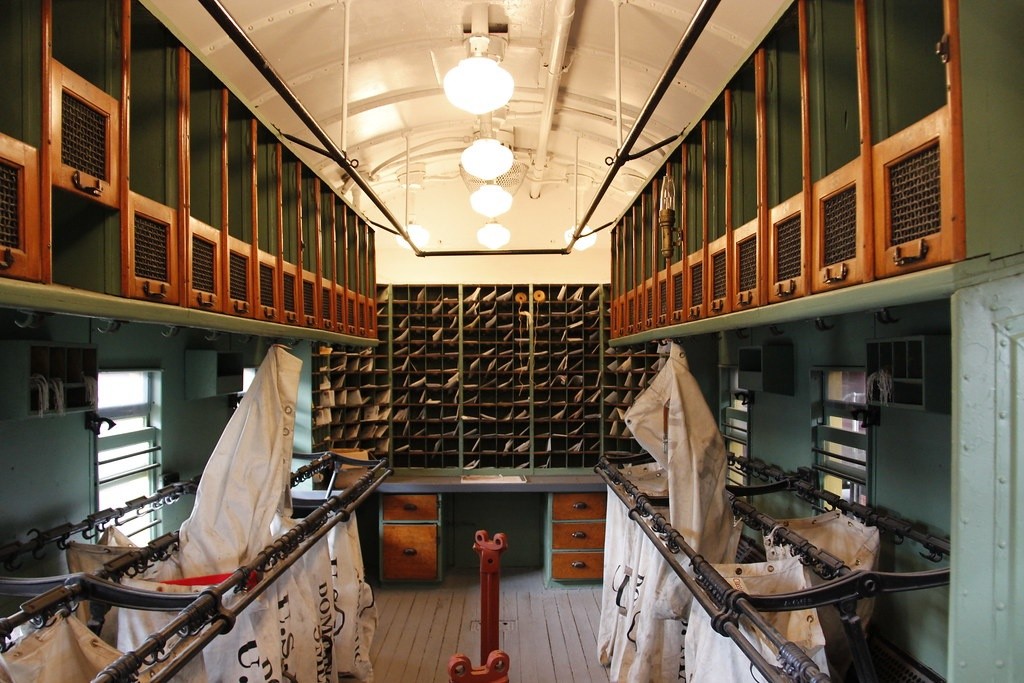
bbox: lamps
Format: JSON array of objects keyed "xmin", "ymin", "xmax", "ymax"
[
  {"xmin": 462, "ymin": 114, "xmax": 517, "ymax": 179},
  {"xmin": 477, "ymin": 220, "xmax": 510, "ymax": 251},
  {"xmin": 398, "ymin": 163, "xmax": 431, "ymax": 251},
  {"xmin": 459, "ymin": 166, "xmax": 530, "ymax": 217},
  {"xmin": 564, "ymin": 174, "xmax": 597, "ymax": 251},
  {"xmin": 442, "ymin": 34, "xmax": 513, "ymax": 116}
]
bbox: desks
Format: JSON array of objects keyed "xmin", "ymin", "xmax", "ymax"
[{"xmin": 374, "ymin": 476, "xmax": 608, "ymax": 589}]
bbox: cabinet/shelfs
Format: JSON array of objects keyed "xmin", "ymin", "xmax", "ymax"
[
  {"xmin": 0, "ymin": 339, "xmax": 100, "ymax": 423},
  {"xmin": 310, "ymin": 283, "xmax": 669, "ymax": 477},
  {"xmin": 595, "ymin": 0, "xmax": 1024, "ymax": 683},
  {"xmin": 0, "ymin": 0, "xmax": 380, "ymax": 683},
  {"xmin": 864, "ymin": 335, "xmax": 949, "ymax": 414}
]
